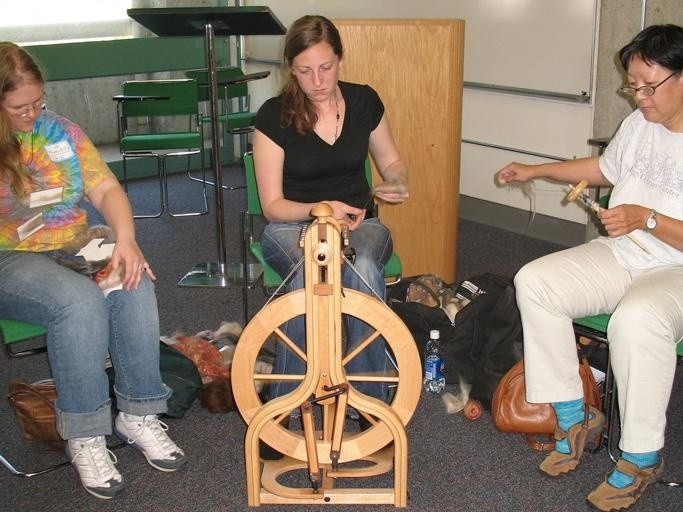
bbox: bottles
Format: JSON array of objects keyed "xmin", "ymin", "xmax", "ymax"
[{"xmin": 423, "ymin": 329, "xmax": 446, "ymax": 392}]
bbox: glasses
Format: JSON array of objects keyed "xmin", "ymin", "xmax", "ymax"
[
  {"xmin": 0, "ymin": 92, "xmax": 47, "ymax": 119},
  {"xmin": 619, "ymin": 69, "xmax": 682, "ymax": 96}
]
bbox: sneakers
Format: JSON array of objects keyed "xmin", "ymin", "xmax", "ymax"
[
  {"xmin": 540, "ymin": 402, "xmax": 606, "ymax": 477},
  {"xmin": 64, "ymin": 434, "xmax": 126, "ymax": 500},
  {"xmin": 113, "ymin": 413, "xmax": 190, "ymax": 473},
  {"xmin": 586, "ymin": 450, "xmax": 664, "ymax": 512},
  {"xmin": 258, "ymin": 383, "xmax": 294, "ymax": 460},
  {"xmin": 359, "ymin": 413, "xmax": 394, "ymax": 448}
]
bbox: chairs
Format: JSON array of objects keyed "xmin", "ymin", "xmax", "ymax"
[{"xmin": 568, "ymin": 196, "xmax": 683, "ymax": 490}]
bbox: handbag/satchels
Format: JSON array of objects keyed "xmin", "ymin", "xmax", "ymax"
[
  {"xmin": 455, "ymin": 272, "xmax": 514, "ymax": 303},
  {"xmin": 491, "ymin": 345, "xmax": 603, "ymax": 452},
  {"xmin": 385, "ymin": 275, "xmax": 455, "ymax": 385},
  {"xmin": 105, "ymin": 339, "xmax": 203, "ymax": 419},
  {"xmin": 7, "ymin": 378, "xmax": 65, "ymax": 447},
  {"xmin": 438, "ymin": 283, "xmax": 524, "ymax": 410}
]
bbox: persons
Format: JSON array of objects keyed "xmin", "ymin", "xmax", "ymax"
[
  {"xmin": 256, "ymin": 13, "xmax": 410, "ymax": 464},
  {"xmin": 0, "ymin": 41, "xmax": 185, "ymax": 500},
  {"xmin": 496, "ymin": 25, "xmax": 681, "ymax": 512}
]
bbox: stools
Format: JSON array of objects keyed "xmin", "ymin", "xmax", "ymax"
[{"xmin": 0, "ymin": 313, "xmax": 137, "ymax": 479}]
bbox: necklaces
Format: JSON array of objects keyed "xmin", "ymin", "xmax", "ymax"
[
  {"xmin": 21, "ymin": 133, "xmax": 35, "ymax": 166},
  {"xmin": 329, "ymin": 93, "xmax": 342, "ymax": 143}
]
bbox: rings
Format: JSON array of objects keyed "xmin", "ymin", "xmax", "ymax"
[{"xmin": 137, "ymin": 267, "xmax": 147, "ymax": 272}]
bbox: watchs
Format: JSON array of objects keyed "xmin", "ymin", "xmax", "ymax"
[{"xmin": 643, "ymin": 207, "xmax": 657, "ymax": 231}]
